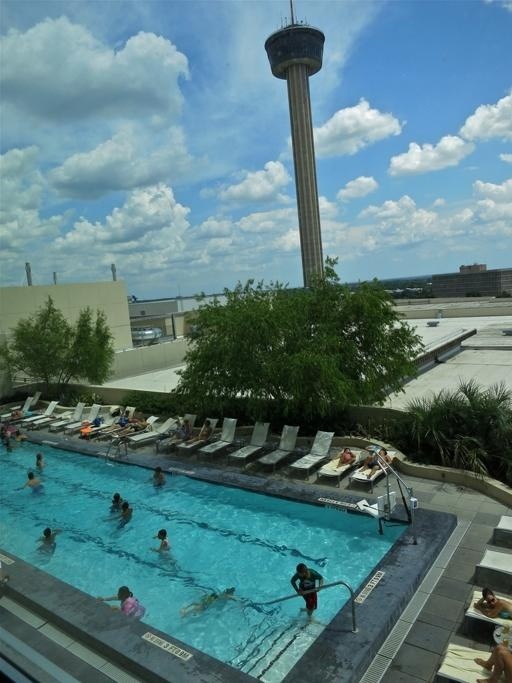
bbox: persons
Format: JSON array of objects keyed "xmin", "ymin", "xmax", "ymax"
[
  {"xmin": 185, "ymin": 419, "xmax": 212, "ymax": 446},
  {"xmin": 36, "ymin": 451, "xmax": 45, "ymax": 468},
  {"xmin": 97, "ymin": 584, "xmax": 147, "ymax": 622},
  {"xmin": 150, "ymin": 528, "xmax": 171, "ymax": 553},
  {"xmin": 0, "ymin": 407, "xmax": 29, "ymax": 453},
  {"xmin": 108, "ymin": 492, "xmax": 122, "ymax": 514},
  {"xmin": 145, "ymin": 465, "xmax": 166, "ymax": 488},
  {"xmin": 291, "ymin": 562, "xmax": 325, "ymax": 620},
  {"xmin": 117, "ymin": 416, "xmax": 153, "ymax": 434},
  {"xmin": 333, "ymin": 446, "xmax": 356, "ymax": 467},
  {"xmin": 35, "ymin": 527, "xmax": 61, "ymax": 555},
  {"xmin": 117, "ymin": 501, "xmax": 133, "ymax": 532},
  {"xmin": 359, "ymin": 448, "xmax": 392, "ymax": 480},
  {"xmin": 175, "ymin": 418, "xmax": 193, "ymax": 439},
  {"xmin": 473, "ymin": 586, "xmax": 511, "ymax": 619},
  {"xmin": 106, "ymin": 408, "xmax": 130, "ymax": 432},
  {"xmin": 472, "ymin": 645, "xmax": 511, "ymax": 683},
  {"xmin": 12, "ymin": 470, "xmax": 44, "ymax": 495},
  {"xmin": 181, "ymin": 585, "xmax": 237, "ymax": 618}
]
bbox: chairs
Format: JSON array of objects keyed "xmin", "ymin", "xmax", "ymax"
[{"xmin": 434, "ymin": 515, "xmax": 512, "ymax": 682}]
